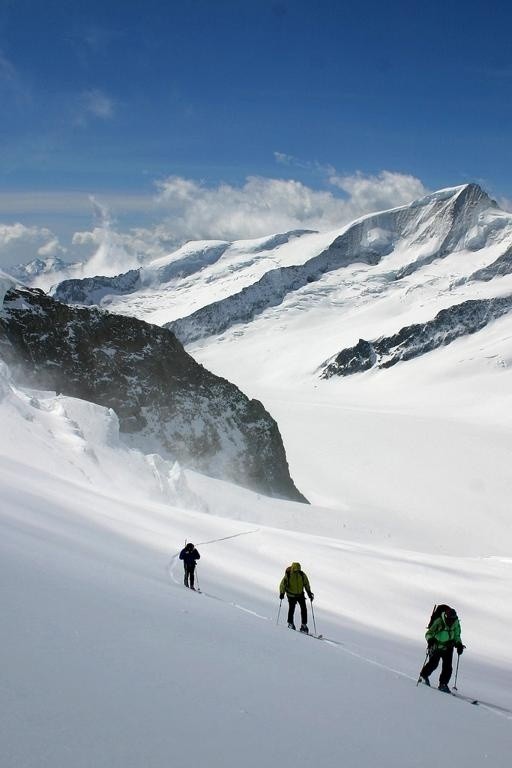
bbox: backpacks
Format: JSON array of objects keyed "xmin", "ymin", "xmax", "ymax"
[
  {"xmin": 285, "ymin": 567, "xmax": 304, "ymax": 587},
  {"xmin": 428, "ymin": 604, "xmax": 452, "ymax": 633}
]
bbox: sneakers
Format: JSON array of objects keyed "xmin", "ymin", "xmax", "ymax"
[
  {"xmin": 420, "ymin": 675, "xmax": 430, "ymax": 686},
  {"xmin": 301, "ymin": 624, "xmax": 308, "ymax": 631},
  {"xmin": 439, "ymin": 685, "xmax": 451, "ymax": 693},
  {"xmin": 288, "ymin": 623, "xmax": 295, "ymax": 630}
]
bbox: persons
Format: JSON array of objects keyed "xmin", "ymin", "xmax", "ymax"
[
  {"xmin": 420, "ymin": 608, "xmax": 463, "ymax": 693},
  {"xmin": 279, "ymin": 562, "xmax": 314, "ymax": 634},
  {"xmin": 179, "ymin": 542, "xmax": 200, "ymax": 591}
]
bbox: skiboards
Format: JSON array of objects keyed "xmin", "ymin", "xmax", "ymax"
[
  {"xmin": 418, "ymin": 678, "xmax": 481, "ymax": 704},
  {"xmin": 288, "ymin": 623, "xmax": 323, "ymax": 640}
]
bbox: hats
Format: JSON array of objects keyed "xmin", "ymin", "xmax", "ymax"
[
  {"xmin": 293, "ymin": 564, "xmax": 301, "ymax": 573},
  {"xmin": 445, "ymin": 608, "xmax": 457, "ymax": 620}
]
